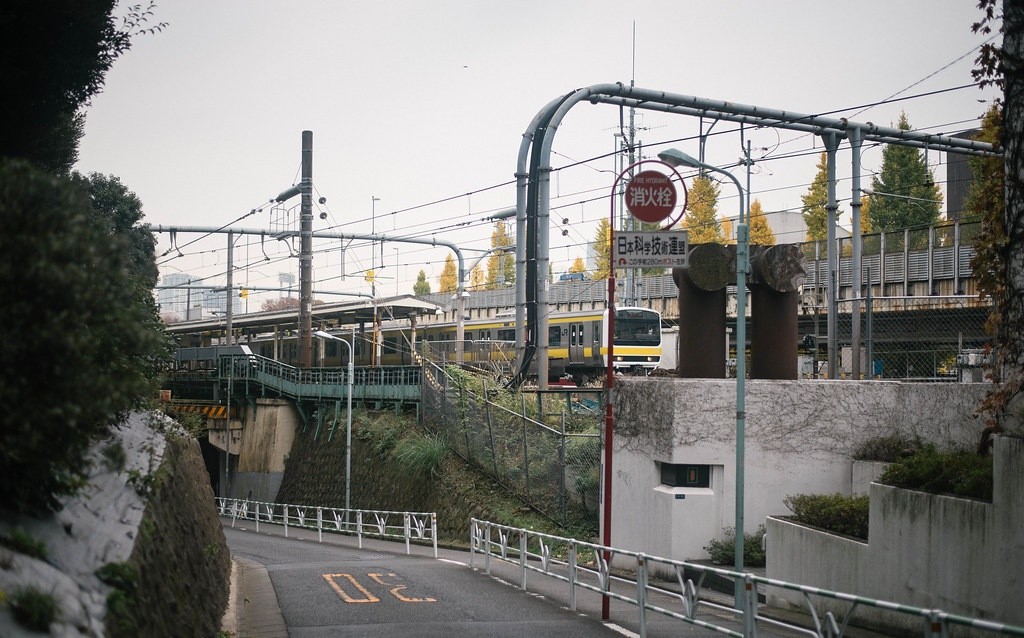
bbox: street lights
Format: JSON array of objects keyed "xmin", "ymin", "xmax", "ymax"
[
  {"xmin": 372, "ymin": 196, "xmax": 381, "ymax": 296},
  {"xmin": 656, "ymin": 148, "xmax": 749, "ymax": 619},
  {"xmin": 614, "ymin": 131, "xmax": 624, "ymax": 231},
  {"xmin": 313, "ymin": 330, "xmax": 353, "ymax": 532}
]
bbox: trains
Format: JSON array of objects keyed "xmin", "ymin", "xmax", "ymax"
[{"xmin": 196, "ymin": 307, "xmax": 662, "ymax": 375}]
